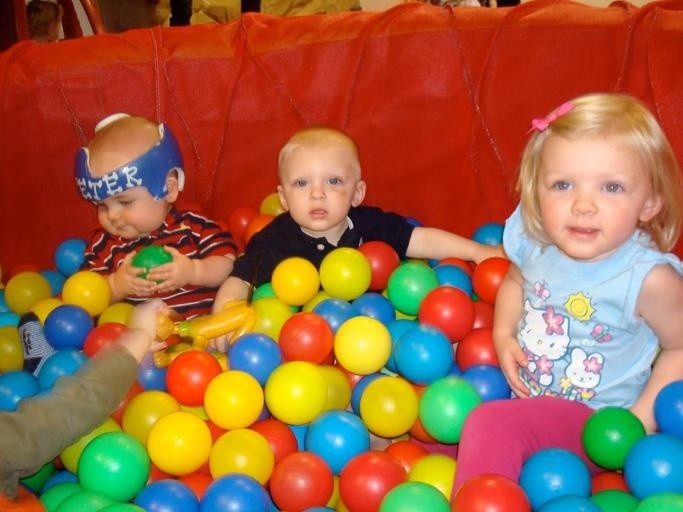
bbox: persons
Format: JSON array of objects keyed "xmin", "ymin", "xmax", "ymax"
[
  {"xmin": 492, "ymin": 92, "xmax": 683, "ymax": 436},
  {"xmin": 25, "ymin": 0, "xmax": 62, "ymax": 45},
  {"xmin": 17, "ymin": 115, "xmax": 239, "ymax": 380},
  {"xmin": 204, "ymin": 127, "xmax": 509, "ymax": 353}
]
